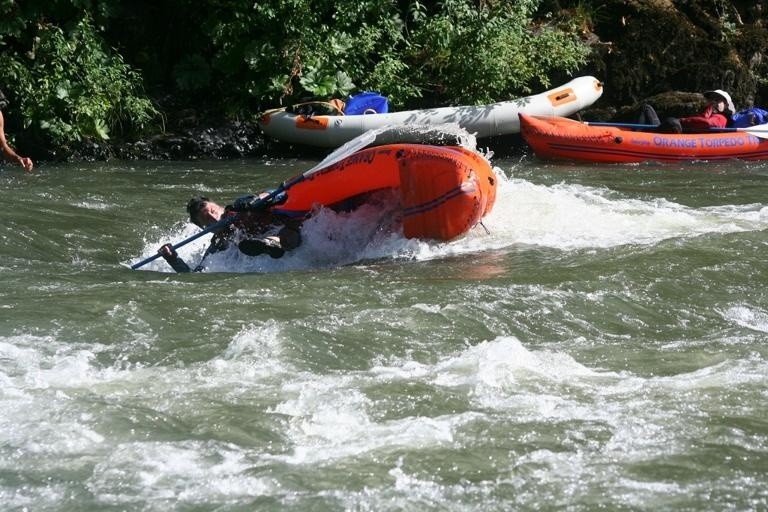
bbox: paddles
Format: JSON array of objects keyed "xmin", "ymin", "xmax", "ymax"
[
  {"xmin": 130, "ymin": 129, "xmax": 376, "ymax": 269},
  {"xmin": 681, "ymin": 124, "xmax": 767, "ymax": 139},
  {"xmin": 532, "ymin": 115, "xmax": 659, "ymax": 127}
]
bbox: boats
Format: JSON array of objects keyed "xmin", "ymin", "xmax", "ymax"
[
  {"xmin": 257, "ymin": 76, "xmax": 607, "ymax": 152},
  {"xmin": 267, "ymin": 142, "xmax": 497, "ymax": 246},
  {"xmin": 515, "ymin": 108, "xmax": 767, "ymax": 164}
]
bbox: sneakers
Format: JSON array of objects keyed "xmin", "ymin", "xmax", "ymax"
[{"xmin": 239, "ymin": 237, "xmax": 285, "ymax": 258}]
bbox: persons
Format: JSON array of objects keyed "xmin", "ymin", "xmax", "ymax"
[
  {"xmin": 159, "ymin": 195, "xmax": 304, "ymax": 273},
  {"xmin": 604, "ymin": 88, "xmax": 736, "ymax": 136},
  {"xmin": 0, "ymin": 90, "xmax": 36, "ymax": 172}
]
